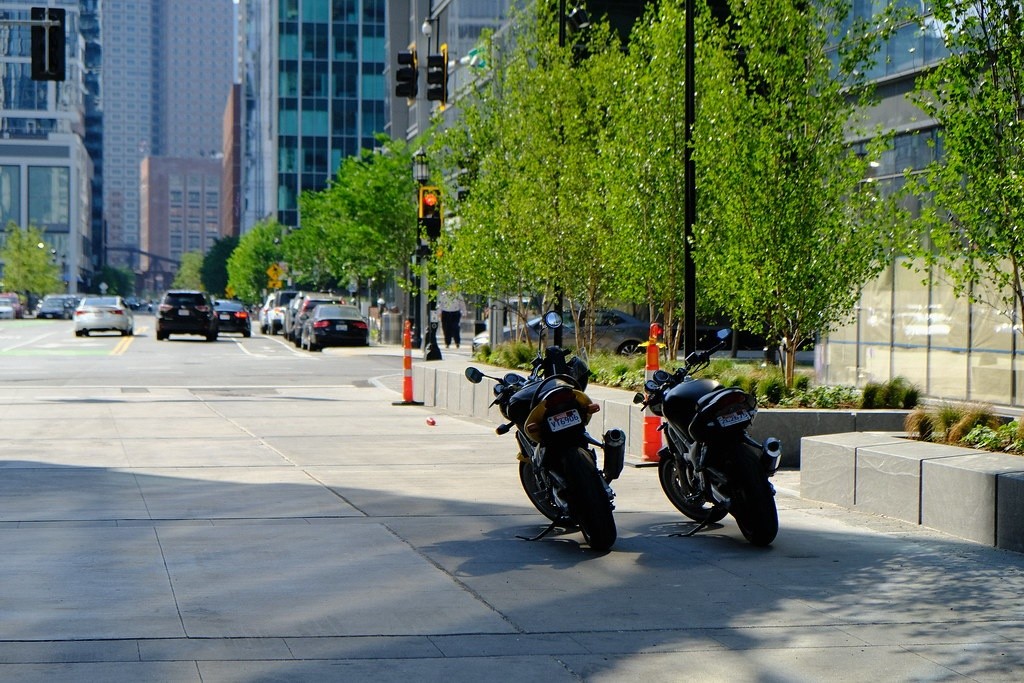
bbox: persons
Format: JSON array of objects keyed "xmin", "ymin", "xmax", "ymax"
[{"xmin": 436, "ymin": 282, "xmax": 467, "ymax": 348}]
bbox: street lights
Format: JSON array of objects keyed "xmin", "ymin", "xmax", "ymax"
[{"xmin": 411, "ymin": 146, "xmax": 427, "ymax": 348}]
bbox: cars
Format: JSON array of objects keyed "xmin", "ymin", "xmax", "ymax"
[
  {"xmin": 37, "ymin": 295, "xmax": 80, "ymax": 319},
  {"xmin": 0, "ymin": 292, "xmax": 22, "ymax": 319},
  {"xmin": 471, "ymin": 310, "xmax": 662, "ymax": 356},
  {"xmin": 73, "ymin": 295, "xmax": 134, "ymax": 335},
  {"xmin": 301, "ymin": 305, "xmax": 369, "ymax": 351},
  {"xmin": 215, "ymin": 299, "xmax": 251, "ymax": 337}
]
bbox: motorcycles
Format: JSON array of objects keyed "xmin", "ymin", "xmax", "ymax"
[
  {"xmin": 466, "ymin": 310, "xmax": 626, "ymax": 551},
  {"xmin": 633, "ymin": 328, "xmax": 782, "ymax": 546}
]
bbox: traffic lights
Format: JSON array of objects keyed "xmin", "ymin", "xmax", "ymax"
[
  {"xmin": 396, "ymin": 43, "xmax": 418, "ymax": 106},
  {"xmin": 421, "ymin": 187, "xmax": 440, "ymax": 240},
  {"xmin": 428, "ymin": 43, "xmax": 448, "ymax": 111}
]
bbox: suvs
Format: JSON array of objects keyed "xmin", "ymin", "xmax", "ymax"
[
  {"xmin": 155, "ymin": 289, "xmax": 219, "ymax": 341},
  {"xmin": 259, "ymin": 291, "xmax": 347, "ymax": 347}
]
